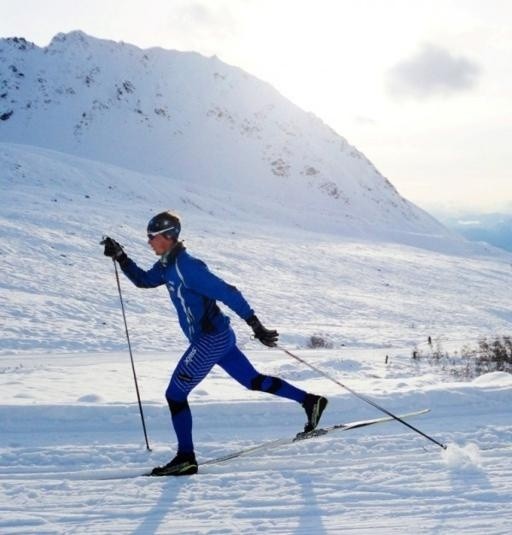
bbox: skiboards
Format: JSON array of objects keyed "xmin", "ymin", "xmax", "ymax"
[{"xmin": 96, "ymin": 407, "xmax": 430, "ymax": 481}]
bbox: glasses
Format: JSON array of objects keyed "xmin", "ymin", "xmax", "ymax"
[{"xmin": 148, "ymin": 225, "xmax": 174, "ymax": 240}]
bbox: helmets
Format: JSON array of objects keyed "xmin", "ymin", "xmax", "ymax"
[{"xmin": 147, "ymin": 213, "xmax": 179, "ymax": 241}]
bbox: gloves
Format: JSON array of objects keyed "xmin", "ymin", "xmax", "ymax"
[
  {"xmin": 99, "ymin": 238, "xmax": 126, "ymax": 261},
  {"xmin": 247, "ymin": 316, "xmax": 278, "ymax": 345}
]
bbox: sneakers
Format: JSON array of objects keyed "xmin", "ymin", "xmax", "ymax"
[
  {"xmin": 303, "ymin": 395, "xmax": 327, "ymax": 431},
  {"xmin": 153, "ymin": 454, "xmax": 197, "ymax": 476}
]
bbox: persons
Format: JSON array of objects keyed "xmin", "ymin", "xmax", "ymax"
[{"xmin": 97, "ymin": 211, "xmax": 331, "ymax": 478}]
